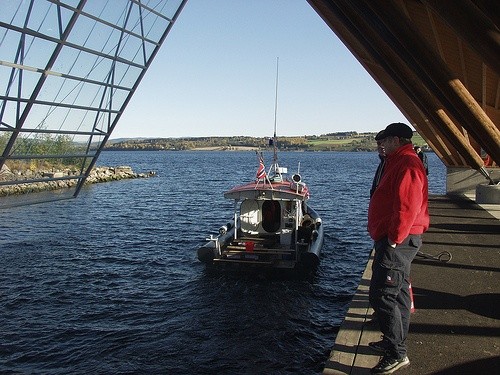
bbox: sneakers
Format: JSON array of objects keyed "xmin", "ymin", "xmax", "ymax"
[
  {"xmin": 372, "ymin": 353, "xmax": 411, "ymax": 375},
  {"xmin": 369, "ymin": 339, "xmax": 386, "ymax": 352}
]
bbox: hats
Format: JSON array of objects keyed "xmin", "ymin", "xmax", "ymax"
[
  {"xmin": 377, "ymin": 123, "xmax": 413, "ymax": 140},
  {"xmin": 375, "ymin": 130, "xmax": 386, "ymax": 140}
]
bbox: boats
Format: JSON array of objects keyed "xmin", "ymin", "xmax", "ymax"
[{"xmin": 196, "ymin": 57, "xmax": 325, "ymax": 279}]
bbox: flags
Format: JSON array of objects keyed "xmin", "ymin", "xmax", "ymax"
[{"xmin": 257, "ymin": 163, "xmax": 266, "ymax": 179}]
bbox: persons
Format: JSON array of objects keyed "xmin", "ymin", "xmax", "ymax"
[
  {"xmin": 413, "ymin": 145, "xmax": 429, "ymax": 176},
  {"xmin": 363, "ymin": 130, "xmax": 386, "ymax": 329},
  {"xmin": 368, "ymin": 122, "xmax": 429, "ymax": 375}
]
